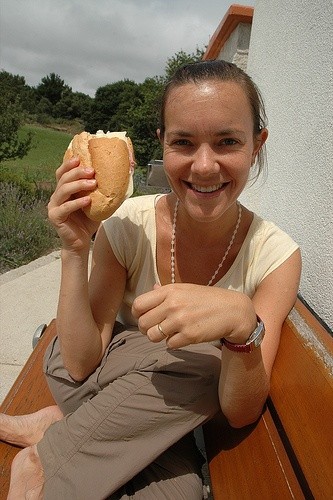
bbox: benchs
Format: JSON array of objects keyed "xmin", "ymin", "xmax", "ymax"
[{"xmin": 0, "ymin": 294, "xmax": 332, "ymax": 500}]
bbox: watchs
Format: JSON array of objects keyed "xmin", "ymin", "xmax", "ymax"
[{"xmin": 217, "ymin": 313, "xmax": 267, "ymax": 354}]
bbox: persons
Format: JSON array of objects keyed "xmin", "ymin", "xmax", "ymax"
[{"xmin": 2, "ymin": 60, "xmax": 303, "ymax": 499}]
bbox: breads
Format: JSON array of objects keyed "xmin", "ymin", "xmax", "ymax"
[{"xmin": 61, "ymin": 131, "xmax": 138, "ymax": 221}]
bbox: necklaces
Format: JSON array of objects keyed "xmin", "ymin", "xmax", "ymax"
[{"xmin": 166, "ymin": 193, "xmax": 243, "ymax": 292}]
